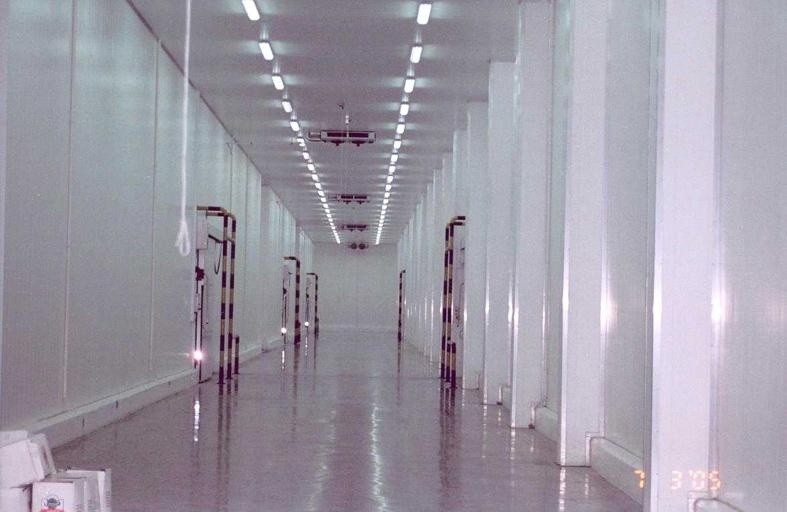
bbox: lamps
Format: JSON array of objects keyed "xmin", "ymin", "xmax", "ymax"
[
  {"xmin": 375, "ymin": 3, "xmax": 432, "ymax": 247},
  {"xmin": 241, "ymin": 0, "xmax": 342, "ymax": 246}
]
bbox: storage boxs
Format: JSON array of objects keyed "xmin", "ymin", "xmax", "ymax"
[{"xmin": 0, "ymin": 429, "xmax": 113, "ymax": 512}]
